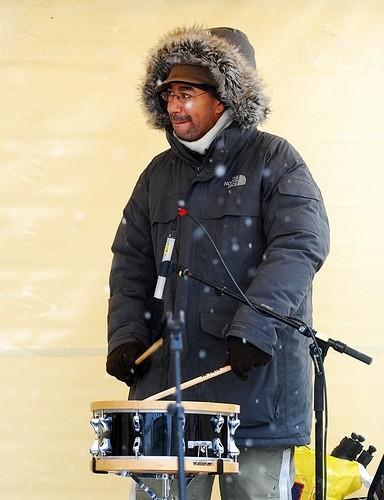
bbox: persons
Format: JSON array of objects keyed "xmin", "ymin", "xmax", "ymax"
[{"xmin": 106, "ymin": 22, "xmax": 331, "ymax": 500}]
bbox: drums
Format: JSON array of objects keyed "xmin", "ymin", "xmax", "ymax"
[{"xmin": 90, "ymin": 401, "xmax": 241, "ymax": 475}]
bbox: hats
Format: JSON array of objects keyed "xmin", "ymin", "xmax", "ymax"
[{"xmin": 154, "ymin": 64, "xmax": 219, "ymax": 93}]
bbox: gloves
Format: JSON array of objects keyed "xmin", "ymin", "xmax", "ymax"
[
  {"xmin": 218, "ymin": 335, "xmax": 272, "ymax": 382},
  {"xmin": 106, "ymin": 342, "xmax": 150, "ymax": 387}
]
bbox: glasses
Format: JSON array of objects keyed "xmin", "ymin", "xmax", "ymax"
[{"xmin": 159, "ymin": 88, "xmax": 214, "ymax": 103}]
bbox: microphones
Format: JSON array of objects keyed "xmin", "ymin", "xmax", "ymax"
[{"xmin": 154, "ymin": 215, "xmax": 180, "ymax": 300}]
